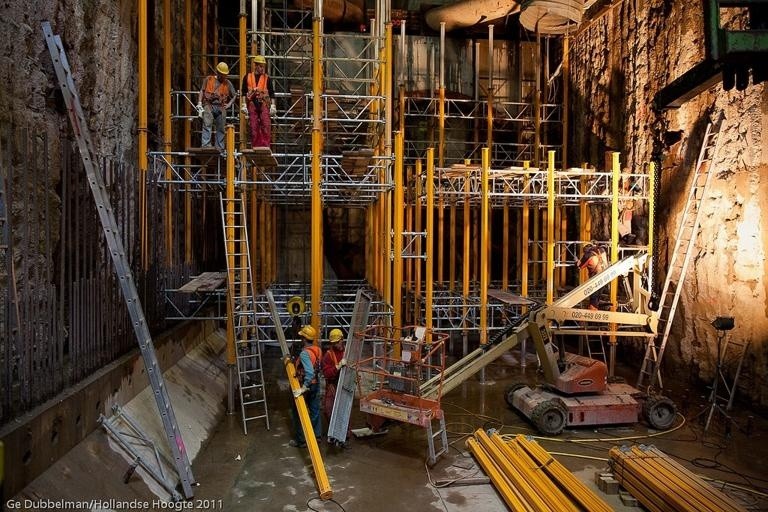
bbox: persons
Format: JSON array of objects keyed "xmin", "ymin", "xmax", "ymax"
[
  {"xmin": 287, "ymin": 325, "xmax": 320, "ymax": 446},
  {"xmin": 197, "ymin": 61, "xmax": 235, "ymax": 148},
  {"xmin": 574, "ymin": 241, "xmax": 609, "ymax": 311},
  {"xmin": 323, "ymin": 329, "xmax": 361, "ymax": 449},
  {"xmin": 629, "ymin": 226, "xmax": 647, "ymax": 288},
  {"xmin": 241, "ymin": 54, "xmax": 275, "ymax": 148}
]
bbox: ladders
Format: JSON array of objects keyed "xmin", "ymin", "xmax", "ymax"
[
  {"xmin": 219, "ymin": 192, "xmax": 270, "ymax": 435},
  {"xmin": 41, "ymin": 18, "xmax": 201, "ymax": 501},
  {"xmin": 636, "ymin": 118, "xmax": 728, "ymax": 395}
]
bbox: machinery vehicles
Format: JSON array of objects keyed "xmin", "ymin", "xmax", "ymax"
[{"xmin": 348, "ymin": 244, "xmax": 676, "ymax": 466}]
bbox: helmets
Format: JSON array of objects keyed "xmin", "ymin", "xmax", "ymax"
[
  {"xmin": 214, "ymin": 60, "xmax": 230, "ymax": 76},
  {"xmin": 296, "ymin": 323, "xmax": 317, "ymax": 341},
  {"xmin": 252, "ymin": 54, "xmax": 268, "ymax": 65},
  {"xmin": 327, "ymin": 327, "xmax": 346, "ymax": 344}
]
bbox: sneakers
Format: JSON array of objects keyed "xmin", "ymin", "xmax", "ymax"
[{"xmin": 288, "ymin": 439, "xmax": 307, "ymax": 448}]
